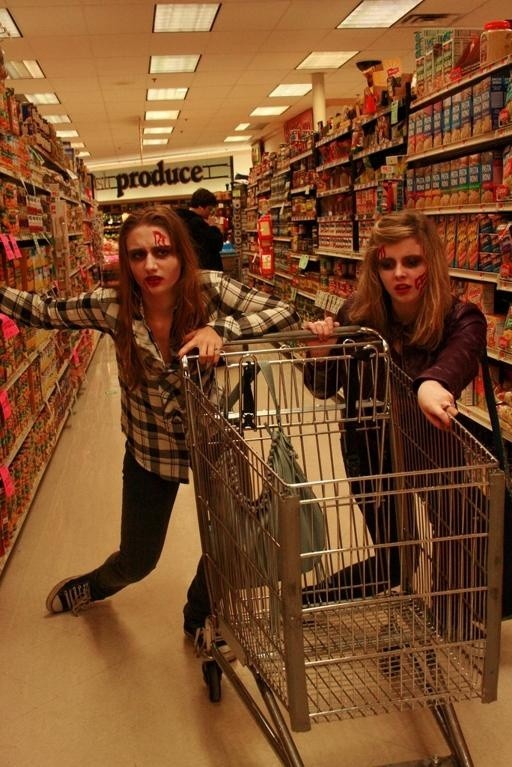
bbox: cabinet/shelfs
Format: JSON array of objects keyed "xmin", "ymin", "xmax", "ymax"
[
  {"xmin": 0, "ymin": 168, "xmax": 105, "ymax": 578},
  {"xmin": 235, "ymin": 55, "xmax": 512, "ymax": 438}
]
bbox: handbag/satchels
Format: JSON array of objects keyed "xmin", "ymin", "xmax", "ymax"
[
  {"xmin": 426, "ymin": 410, "xmax": 512, "ymax": 624},
  {"xmin": 204, "ymin": 358, "xmax": 328, "ymax": 584}
]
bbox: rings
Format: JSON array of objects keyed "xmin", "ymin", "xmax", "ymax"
[{"xmin": 443, "ymin": 405, "xmax": 451, "ymax": 411}]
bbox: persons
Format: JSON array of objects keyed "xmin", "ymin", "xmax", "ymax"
[
  {"xmin": 294, "ymin": 204, "xmax": 511, "ymax": 660},
  {"xmin": 175, "ymin": 187, "xmax": 225, "ymax": 273},
  {"xmin": 1, "ymin": 203, "xmax": 298, "ymax": 663}
]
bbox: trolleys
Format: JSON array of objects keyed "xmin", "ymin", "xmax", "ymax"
[{"xmin": 182, "ymin": 325, "xmax": 506, "ymax": 767}]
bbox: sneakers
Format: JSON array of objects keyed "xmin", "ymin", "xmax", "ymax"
[
  {"xmin": 44, "ymin": 569, "xmax": 114, "ymax": 616},
  {"xmin": 181, "ymin": 616, "xmax": 237, "ymax": 665}
]
